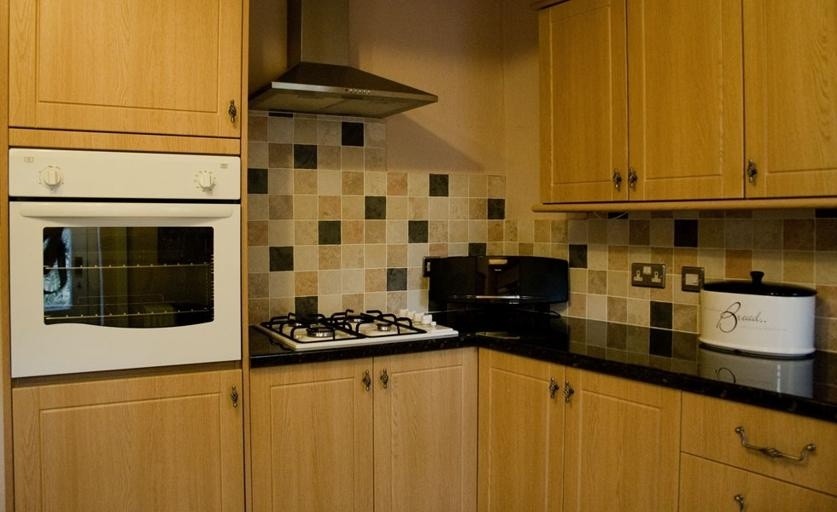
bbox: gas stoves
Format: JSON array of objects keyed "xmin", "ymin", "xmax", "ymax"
[{"xmin": 257, "ymin": 304, "xmax": 458, "ymax": 353}]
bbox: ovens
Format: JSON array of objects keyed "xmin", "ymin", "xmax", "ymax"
[{"xmin": 9, "ymin": 200, "xmax": 242, "ymax": 381}]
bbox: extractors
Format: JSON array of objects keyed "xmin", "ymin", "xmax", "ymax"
[{"xmin": 248, "ymin": 1, "xmax": 439, "ymax": 121}]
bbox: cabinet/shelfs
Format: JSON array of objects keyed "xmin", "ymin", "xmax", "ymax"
[
  {"xmin": 12, "ymin": 369, "xmax": 244, "ymax": 512},
  {"xmin": 248, "ymin": 348, "xmax": 478, "ymax": 512},
  {"xmin": 6, "ymin": 0, "xmax": 245, "ymax": 140},
  {"xmin": 681, "ymin": 391, "xmax": 835, "ymax": 511},
  {"xmin": 478, "ymin": 348, "xmax": 680, "ymax": 511},
  {"xmin": 540, "ymin": 0, "xmax": 744, "ymax": 203},
  {"xmin": 744, "ymin": 1, "xmax": 837, "ymax": 201}
]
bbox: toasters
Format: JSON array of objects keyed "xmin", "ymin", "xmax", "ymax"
[{"xmin": 699, "ymin": 272, "xmax": 817, "ymax": 360}]
